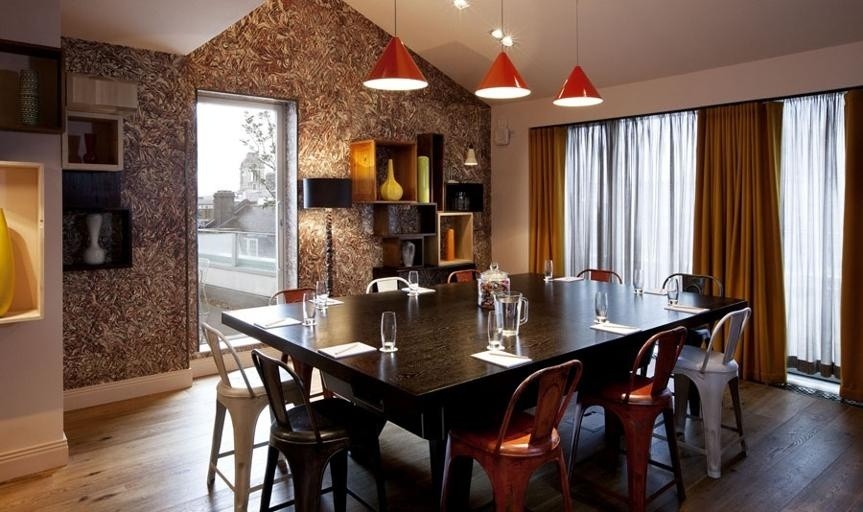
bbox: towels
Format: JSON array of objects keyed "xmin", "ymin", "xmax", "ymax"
[
  {"xmin": 308, "ymin": 298, "xmax": 344, "ymax": 306},
  {"xmin": 401, "ymin": 285, "xmax": 435, "ymax": 296}
]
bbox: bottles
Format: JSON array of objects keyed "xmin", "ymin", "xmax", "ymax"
[{"xmin": 477, "ymin": 264, "xmax": 511, "ymax": 309}]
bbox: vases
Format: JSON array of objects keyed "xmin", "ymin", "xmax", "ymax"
[{"xmin": 83, "ymin": 214, "xmax": 106, "ymax": 264}]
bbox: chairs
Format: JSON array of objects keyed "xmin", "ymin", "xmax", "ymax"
[
  {"xmin": 269, "ymin": 286, "xmax": 335, "ymax": 406},
  {"xmin": 198, "ymin": 258, "xmax": 209, "ymax": 314},
  {"xmin": 198, "ymin": 321, "xmax": 388, "ymax": 512},
  {"xmin": 365, "ymin": 276, "xmax": 410, "ymax": 295}
]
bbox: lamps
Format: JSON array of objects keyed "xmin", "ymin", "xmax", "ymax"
[
  {"xmin": 552, "ymin": 0, "xmax": 604, "ymax": 107},
  {"xmin": 302, "ymin": 178, "xmax": 354, "ymax": 298},
  {"xmin": 474, "ymin": 0, "xmax": 532, "ymax": 100},
  {"xmin": 365, "ymin": 5, "xmax": 429, "ymax": 92}
]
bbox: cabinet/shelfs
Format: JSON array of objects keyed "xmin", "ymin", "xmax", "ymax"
[
  {"xmin": 350, "ymin": 133, "xmax": 476, "ymax": 276},
  {"xmin": 0, "ymin": 160, "xmax": 46, "ymax": 325},
  {"xmin": 63, "ymin": 207, "xmax": 132, "ymax": 272}
]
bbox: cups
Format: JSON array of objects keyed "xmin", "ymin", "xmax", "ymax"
[
  {"xmin": 667, "ymin": 278, "xmax": 681, "ymax": 305},
  {"xmin": 493, "ymin": 292, "xmax": 528, "ymax": 337},
  {"xmin": 381, "ymin": 311, "xmax": 397, "ymax": 350},
  {"xmin": 409, "ymin": 271, "xmax": 418, "ymax": 294},
  {"xmin": 302, "ymin": 292, "xmax": 315, "ymax": 326},
  {"xmin": 594, "ymin": 291, "xmax": 608, "ymax": 323},
  {"xmin": 545, "ymin": 260, "xmax": 553, "ymax": 279},
  {"xmin": 316, "ymin": 280, "xmax": 328, "ymax": 309},
  {"xmin": 487, "ymin": 309, "xmax": 504, "ymax": 348},
  {"xmin": 633, "ymin": 270, "xmax": 645, "ymax": 294}
]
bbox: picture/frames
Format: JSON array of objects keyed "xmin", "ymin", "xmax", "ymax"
[
  {"xmin": 0, "ymin": 38, "xmax": 66, "ymax": 134},
  {"xmin": 62, "ymin": 109, "xmax": 124, "ymax": 172}
]
